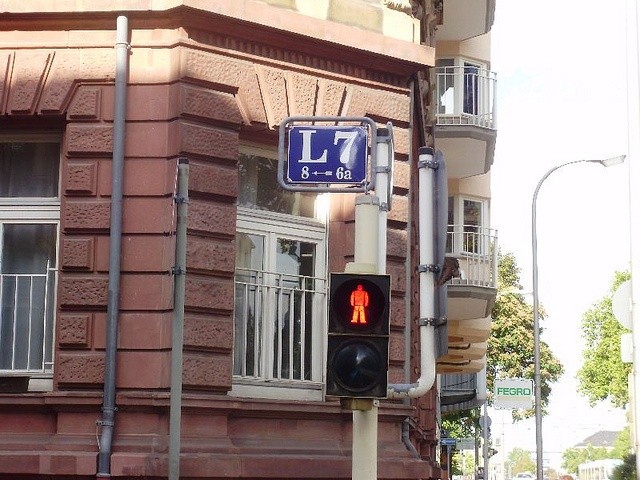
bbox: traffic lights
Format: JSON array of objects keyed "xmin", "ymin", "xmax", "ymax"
[{"xmin": 325, "ymin": 272, "xmax": 391, "ymax": 400}]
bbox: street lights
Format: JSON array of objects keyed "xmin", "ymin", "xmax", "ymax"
[{"xmin": 530, "ymin": 155, "xmax": 625, "ymax": 479}]
